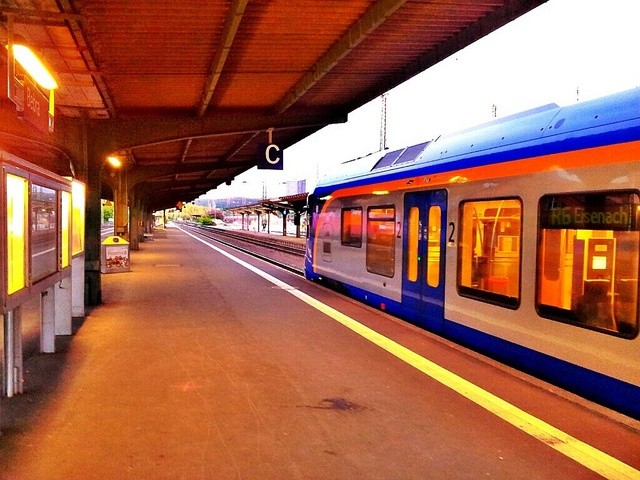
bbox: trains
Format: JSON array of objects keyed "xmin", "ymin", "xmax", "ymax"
[{"xmin": 305, "ymin": 86, "xmax": 640, "ymax": 421}]
